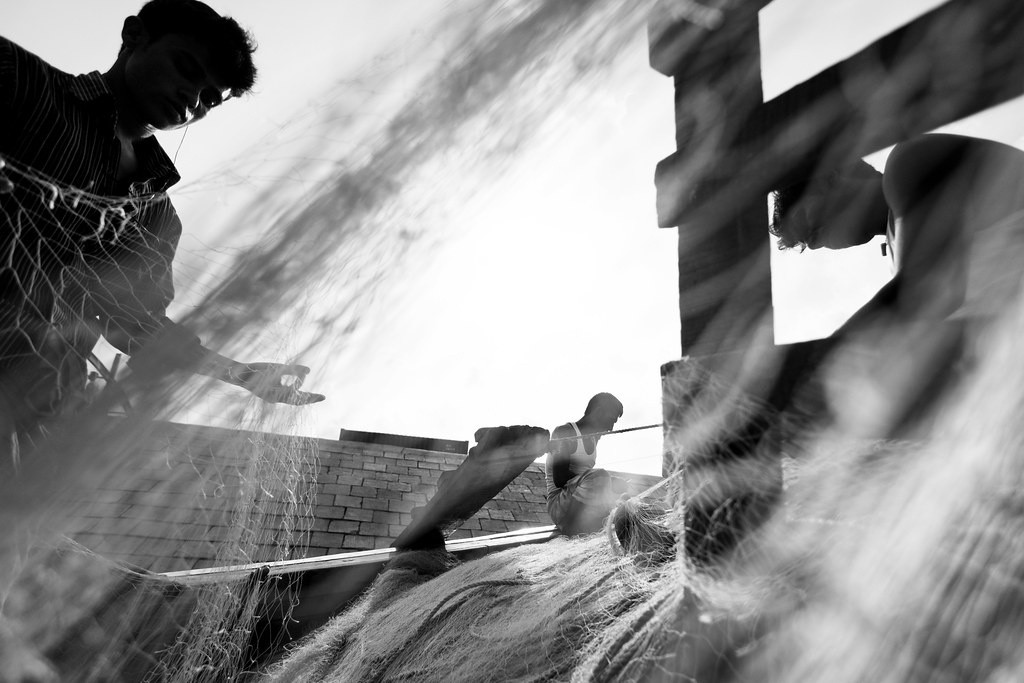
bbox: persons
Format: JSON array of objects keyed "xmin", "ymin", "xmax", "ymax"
[
  {"xmin": 0, "ymin": 0, "xmax": 326, "ymax": 460},
  {"xmin": 544, "ymin": 392, "xmax": 637, "ymax": 539}
]
